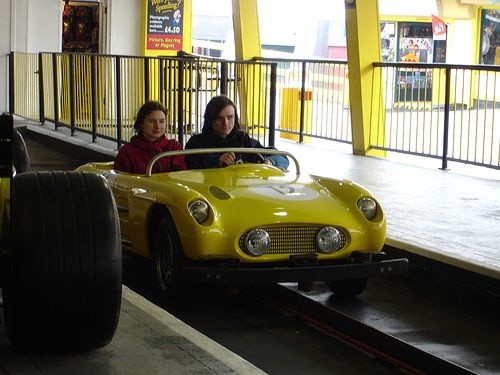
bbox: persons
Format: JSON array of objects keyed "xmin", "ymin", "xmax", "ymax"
[
  {"xmin": 183, "ymin": 95, "xmax": 290, "ymax": 173},
  {"xmin": 114, "ymin": 102, "xmax": 188, "ymax": 172}
]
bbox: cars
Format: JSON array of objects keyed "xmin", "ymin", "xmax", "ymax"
[{"xmin": 74, "ymin": 146, "xmax": 389, "ymax": 298}]
